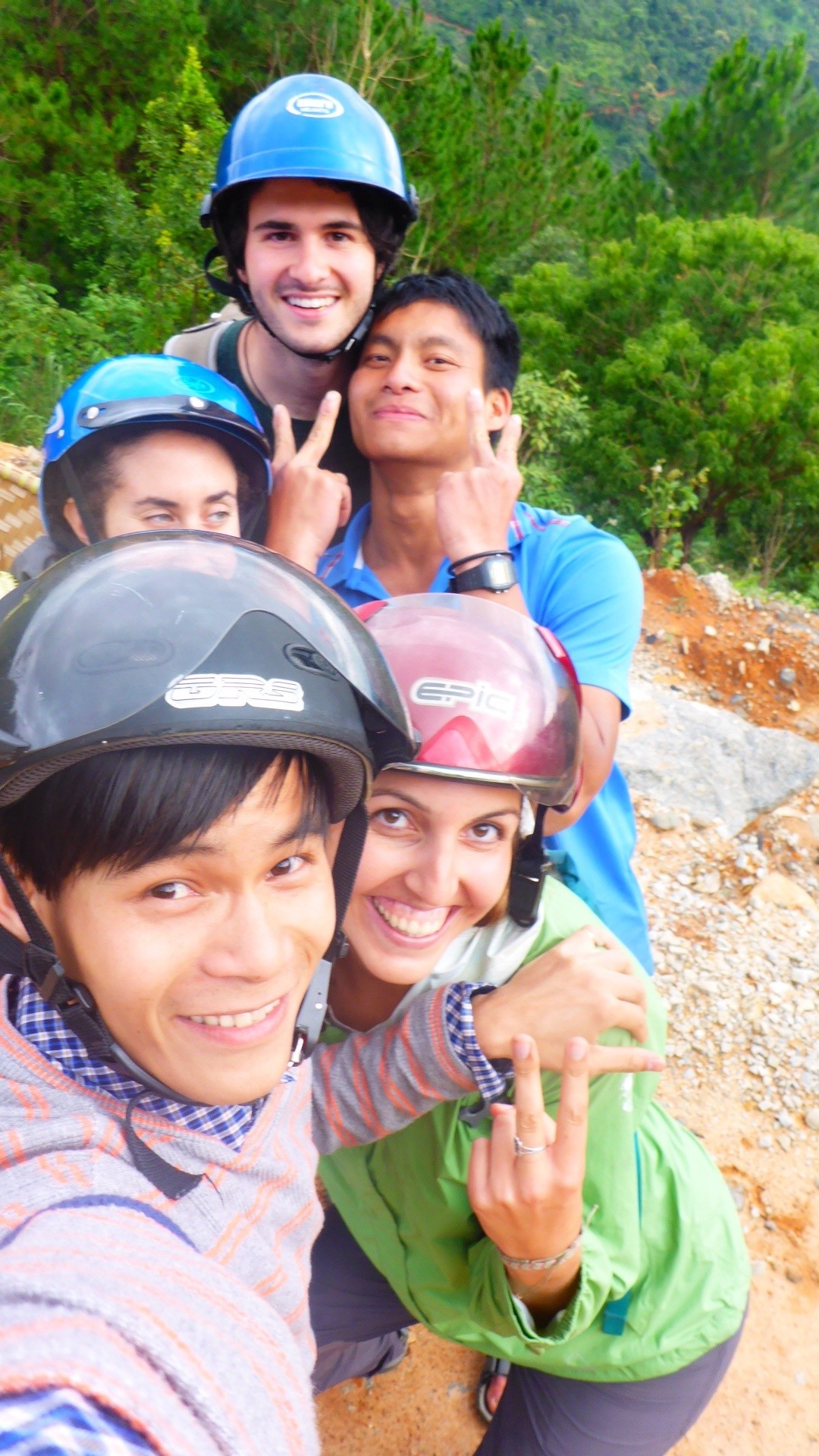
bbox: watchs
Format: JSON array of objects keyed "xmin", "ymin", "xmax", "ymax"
[{"xmin": 449, "ymin": 554, "xmax": 518, "ymax": 594}]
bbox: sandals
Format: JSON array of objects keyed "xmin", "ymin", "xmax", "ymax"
[{"xmin": 475, "ymin": 1355, "xmax": 511, "ymax": 1421}]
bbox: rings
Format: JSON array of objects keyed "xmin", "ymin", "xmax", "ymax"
[{"xmin": 512, "ymin": 1137, "xmax": 544, "ymax": 1154}]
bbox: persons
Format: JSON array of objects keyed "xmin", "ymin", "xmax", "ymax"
[{"xmin": 0, "ymin": 73, "xmax": 751, "ymax": 1456}]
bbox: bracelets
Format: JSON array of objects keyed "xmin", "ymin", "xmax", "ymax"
[
  {"xmin": 498, "ymin": 1205, "xmax": 599, "ymax": 1270},
  {"xmin": 447, "ymin": 551, "xmax": 515, "ymax": 575}
]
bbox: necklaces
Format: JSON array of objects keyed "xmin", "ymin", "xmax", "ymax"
[{"xmin": 245, "ymin": 317, "xmax": 274, "ymax": 412}]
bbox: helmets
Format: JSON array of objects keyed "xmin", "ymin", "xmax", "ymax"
[
  {"xmin": 38, "ymin": 353, "xmax": 273, "ymax": 549},
  {"xmin": 346, "ymin": 593, "xmax": 582, "ymax": 789},
  {"xmin": 199, "ymin": 73, "xmax": 418, "ymax": 272},
  {"xmin": 1, "ymin": 529, "xmax": 414, "ymax": 818}
]
